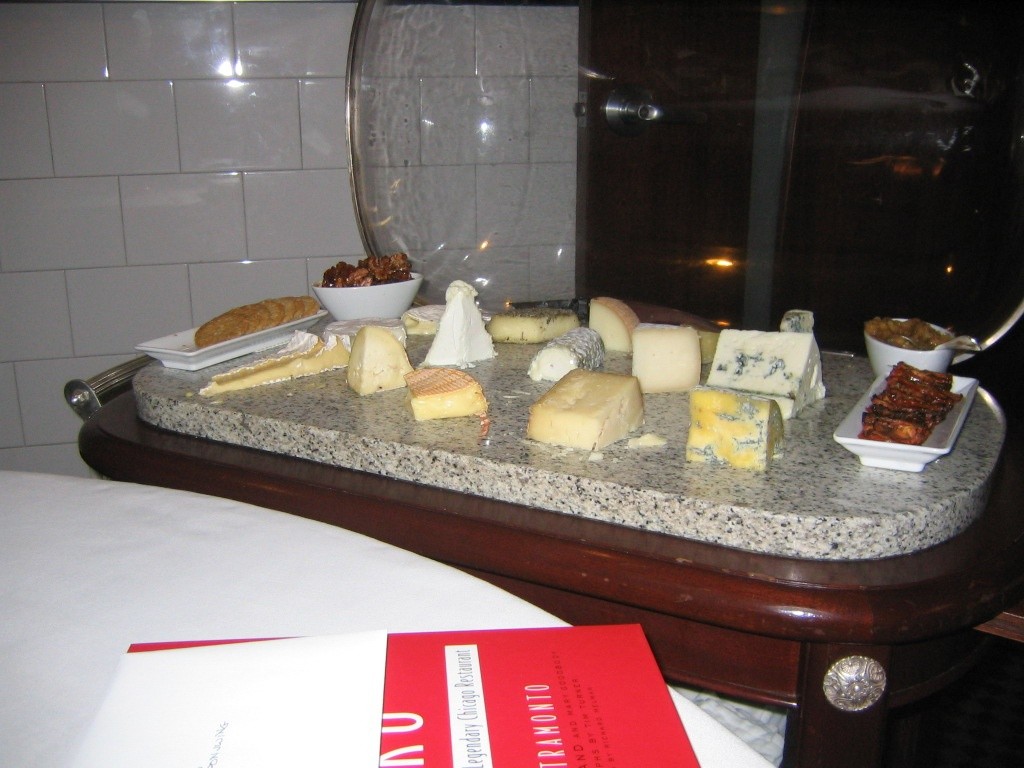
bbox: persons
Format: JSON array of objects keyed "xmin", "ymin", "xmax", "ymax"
[{"xmin": 844, "ymin": 125, "xmax": 980, "ymax": 216}]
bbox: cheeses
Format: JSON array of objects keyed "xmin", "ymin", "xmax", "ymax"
[
  {"xmin": 399, "ymin": 280, "xmax": 826, "ymax": 471},
  {"xmin": 199, "ymin": 317, "xmax": 416, "ymax": 398}
]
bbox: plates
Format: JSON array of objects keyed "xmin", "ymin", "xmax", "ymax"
[
  {"xmin": 833, "ymin": 369, "xmax": 980, "ymax": 472},
  {"xmin": 134, "ymin": 308, "xmax": 328, "ymax": 364}
]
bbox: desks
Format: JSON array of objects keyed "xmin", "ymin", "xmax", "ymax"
[
  {"xmin": 0, "ymin": 469, "xmax": 775, "ymax": 768},
  {"xmin": 77, "ymin": 394, "xmax": 1023, "ymax": 768}
]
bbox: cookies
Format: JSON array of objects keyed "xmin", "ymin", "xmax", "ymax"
[{"xmin": 194, "ymin": 296, "xmax": 319, "ymax": 346}]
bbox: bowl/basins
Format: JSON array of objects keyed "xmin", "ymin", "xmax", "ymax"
[
  {"xmin": 310, "ymin": 273, "xmax": 423, "ymax": 319},
  {"xmin": 864, "ymin": 316, "xmax": 955, "ymax": 377}
]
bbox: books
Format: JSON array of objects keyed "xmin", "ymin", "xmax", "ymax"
[{"xmin": 124, "ymin": 621, "xmax": 700, "ymax": 768}]
